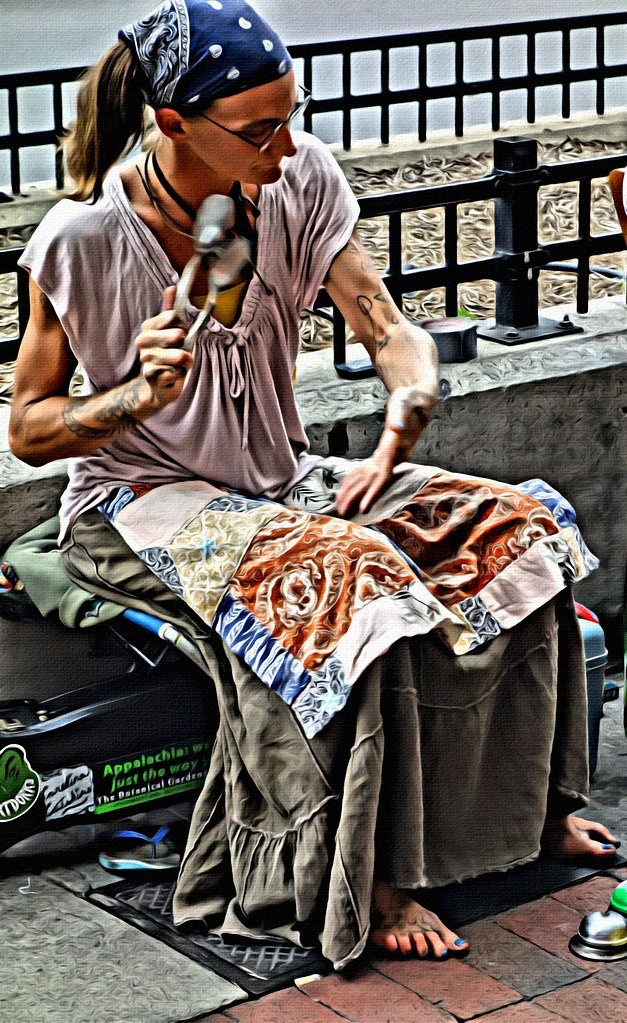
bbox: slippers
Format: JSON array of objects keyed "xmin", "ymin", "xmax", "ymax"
[{"xmin": 99, "ymin": 827, "xmax": 181, "ymax": 878}]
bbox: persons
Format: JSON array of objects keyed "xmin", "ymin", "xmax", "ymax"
[{"xmin": 9, "ymin": 1, "xmax": 620, "ymax": 968}]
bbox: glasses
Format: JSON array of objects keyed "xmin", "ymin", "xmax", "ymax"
[{"xmin": 197, "ymin": 82, "xmax": 312, "ymax": 153}]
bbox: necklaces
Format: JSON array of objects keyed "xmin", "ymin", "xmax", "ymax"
[{"xmin": 136, "ymin": 147, "xmax": 261, "ymax": 242}]
